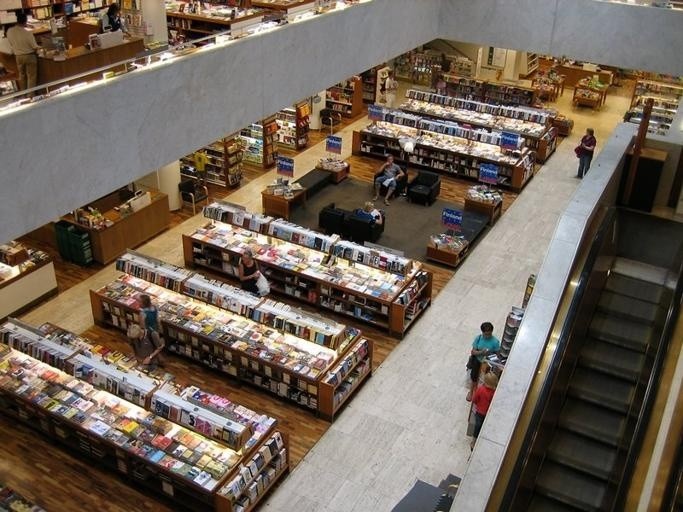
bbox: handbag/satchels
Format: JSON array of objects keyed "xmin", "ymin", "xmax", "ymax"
[
  {"xmin": 466, "ymin": 355, "xmax": 477, "ymax": 372},
  {"xmin": 574, "ymin": 146, "xmax": 583, "ymax": 158}
]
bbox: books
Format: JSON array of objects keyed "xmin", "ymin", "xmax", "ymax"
[
  {"xmin": 179, "ymin": 129, "xmax": 244, "ymax": 188},
  {"xmin": 1, "ymin": 240, "xmax": 47, "ymax": 285},
  {"xmin": 242, "ymin": 100, "xmax": 310, "ymax": 165},
  {"xmin": 1, "ymin": 1, "xmax": 114, "ymax": 33},
  {"xmin": 0, "ymin": 316, "xmax": 288, "ymax": 512},
  {"xmin": 191, "ymin": 199, "xmax": 427, "ymax": 324},
  {"xmin": 409, "ymin": 52, "xmax": 443, "ymax": 82},
  {"xmin": 165, "ymin": 0, "xmax": 204, "ymax": 47},
  {"xmin": 323, "ymin": 79, "xmax": 355, "ymax": 116},
  {"xmin": 622, "ymin": 69, "xmax": 683, "ymax": 134},
  {"xmin": 102, "ymin": 252, "xmax": 370, "ymax": 411},
  {"xmin": 359, "ymin": 73, "xmax": 567, "ymax": 189}
]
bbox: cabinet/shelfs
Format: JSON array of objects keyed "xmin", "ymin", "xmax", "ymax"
[
  {"xmin": 1, "ymin": 316, "xmax": 290, "ymax": 510},
  {"xmin": 353, "ymin": 50, "xmax": 680, "ymax": 193},
  {"xmin": 1, "ymin": 1, "xmax": 329, "ymax": 105},
  {"xmin": 261, "ymin": 156, "xmax": 350, "ymax": 220},
  {"xmin": 426, "ymin": 185, "xmax": 504, "ymax": 268},
  {"xmin": 176, "ymin": 74, "xmax": 363, "ymax": 192},
  {"xmin": 182, "ymin": 200, "xmax": 430, "ymax": 341},
  {"xmin": 90, "ymin": 247, "xmax": 375, "ymax": 421},
  {"xmin": 0, "ymin": 179, "xmax": 169, "ymax": 322}
]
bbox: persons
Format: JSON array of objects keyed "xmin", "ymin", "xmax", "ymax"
[
  {"xmin": 464, "ymin": 372, "xmax": 498, "ymax": 452},
  {"xmin": 575, "ymin": 128, "xmax": 597, "ymax": 180},
  {"xmin": 373, "ymin": 154, "xmax": 406, "ymax": 207},
  {"xmin": 6, "ymin": 12, "xmax": 37, "ymax": 98},
  {"xmin": 464, "ymin": 322, "xmax": 499, "ymax": 402},
  {"xmin": 101, "ymin": 2, "xmax": 133, "ymax": 38}
]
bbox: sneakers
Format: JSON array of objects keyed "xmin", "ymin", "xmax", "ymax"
[
  {"xmin": 372, "ymin": 194, "xmax": 380, "ymax": 201},
  {"xmin": 383, "ymin": 198, "xmax": 391, "ymax": 207}
]
